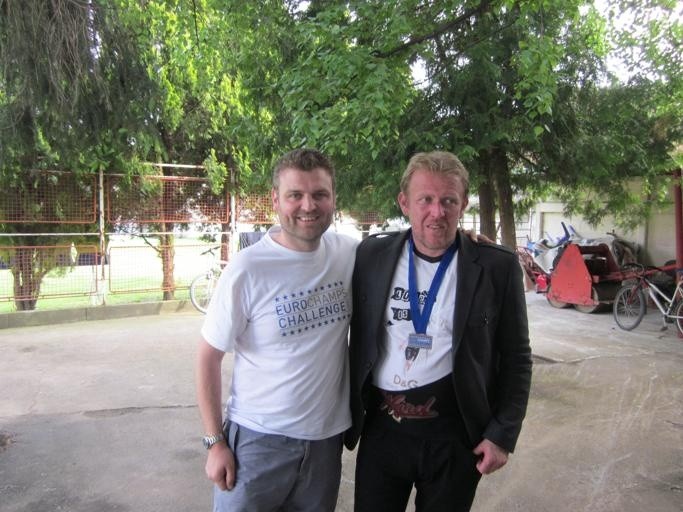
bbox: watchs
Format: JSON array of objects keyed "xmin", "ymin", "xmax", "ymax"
[{"xmin": 201, "ymin": 433, "xmax": 225, "ymax": 450}]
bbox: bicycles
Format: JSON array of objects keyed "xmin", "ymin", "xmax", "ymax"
[
  {"xmin": 613, "ymin": 262, "xmax": 683, "ymax": 337},
  {"xmin": 190, "ymin": 246, "xmax": 233, "ymax": 314}
]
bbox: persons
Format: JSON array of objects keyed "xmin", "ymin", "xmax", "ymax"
[
  {"xmin": 341, "ymin": 149, "xmax": 532, "ymax": 512},
  {"xmin": 198, "ymin": 150, "xmax": 495, "ymax": 511}
]
